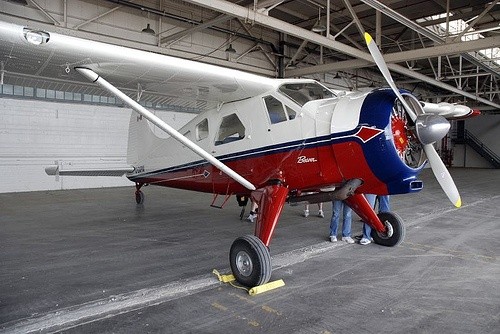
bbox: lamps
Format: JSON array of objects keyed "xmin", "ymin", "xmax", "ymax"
[
  {"xmin": 333, "ymin": 72, "xmax": 341, "ymax": 79},
  {"xmin": 312, "ymin": 7, "xmax": 326, "ymax": 31},
  {"xmin": 411, "ymin": 60, "xmax": 425, "ymax": 69},
  {"xmin": 368, "ymin": 81, "xmax": 376, "ymax": 88},
  {"xmin": 225, "ymin": 19, "xmax": 236, "ymax": 54},
  {"xmin": 141, "ymin": 12, "xmax": 156, "ymax": 35},
  {"xmin": 287, "ymin": 61, "xmax": 297, "ymax": 69},
  {"xmin": 418, "ymin": 96, "xmax": 424, "ymax": 102}
]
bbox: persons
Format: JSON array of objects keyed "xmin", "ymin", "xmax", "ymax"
[
  {"xmin": 247, "ymin": 201, "xmax": 258, "ymax": 219},
  {"xmin": 329, "ymin": 201, "xmax": 354, "ymax": 244},
  {"xmin": 305, "ymin": 203, "xmax": 324, "ymax": 217},
  {"xmin": 360, "ymin": 194, "xmax": 389, "ymax": 245},
  {"xmin": 449, "ymin": 139, "xmax": 457, "ymax": 168}
]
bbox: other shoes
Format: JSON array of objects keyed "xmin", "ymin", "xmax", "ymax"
[
  {"xmin": 331, "ymin": 237, "xmax": 338, "ymax": 242},
  {"xmin": 318, "ymin": 210, "xmax": 324, "ymax": 217},
  {"xmin": 304, "ymin": 210, "xmax": 309, "ymax": 217},
  {"xmin": 342, "ymin": 237, "xmax": 354, "ymax": 244},
  {"xmin": 246, "ymin": 211, "xmax": 258, "ymax": 222},
  {"xmin": 355, "ymin": 234, "xmax": 374, "ymax": 245}
]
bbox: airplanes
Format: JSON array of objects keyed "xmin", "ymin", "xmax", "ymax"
[{"xmin": 44, "ymin": 31, "xmax": 481, "ymax": 290}]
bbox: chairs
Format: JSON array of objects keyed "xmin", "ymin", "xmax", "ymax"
[{"xmin": 269, "ymin": 112, "xmax": 283, "ymax": 124}]
